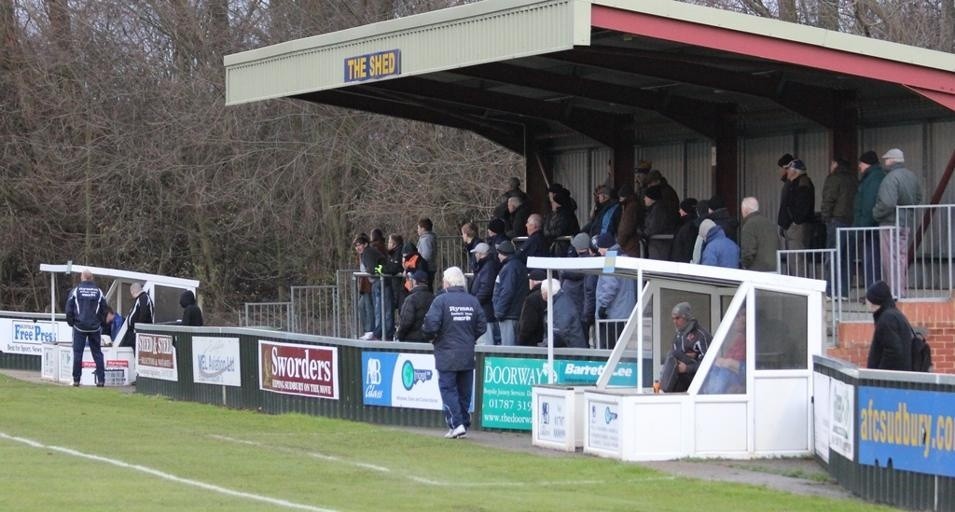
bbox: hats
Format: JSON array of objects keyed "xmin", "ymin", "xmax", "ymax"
[
  {"xmin": 681, "ymin": 196, "xmax": 724, "ymax": 212},
  {"xmin": 572, "ymin": 232, "xmax": 616, "ymax": 249},
  {"xmin": 470, "ymin": 219, "xmax": 514, "ymax": 253},
  {"xmin": 672, "ymin": 302, "xmax": 692, "ymax": 319},
  {"xmin": 777, "ymin": 154, "xmax": 805, "ymax": 170},
  {"xmin": 867, "ymin": 281, "xmax": 890, "ymax": 304},
  {"xmin": 644, "ymin": 169, "xmax": 662, "ymax": 199},
  {"xmin": 860, "ymin": 148, "xmax": 902, "ymax": 164}
]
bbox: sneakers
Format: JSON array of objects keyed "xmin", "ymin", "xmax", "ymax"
[{"xmin": 444, "ymin": 424, "xmax": 468, "ymax": 439}]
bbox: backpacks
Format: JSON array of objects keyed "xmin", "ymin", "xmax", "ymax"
[{"xmin": 910, "ymin": 327, "xmax": 931, "ymax": 371}]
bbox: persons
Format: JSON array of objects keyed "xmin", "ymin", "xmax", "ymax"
[
  {"xmin": 65, "ymin": 272, "xmax": 109, "ymax": 387},
  {"xmin": 852, "ymin": 151, "xmax": 886, "ymax": 305},
  {"xmin": 653, "ymin": 299, "xmax": 714, "ymax": 393},
  {"xmin": 778, "ymin": 153, "xmax": 815, "ymax": 278},
  {"xmin": 127, "ymin": 282, "xmax": 154, "ymax": 386},
  {"xmin": 740, "ymin": 197, "xmax": 779, "ymax": 272},
  {"xmin": 701, "ymin": 309, "xmax": 778, "ymax": 392},
  {"xmin": 461, "ymin": 158, "xmax": 741, "ymax": 347},
  {"xmin": 174, "ymin": 291, "xmax": 205, "ymax": 325},
  {"xmin": 86, "ymin": 303, "xmax": 125, "ymax": 347},
  {"xmin": 872, "ymin": 148, "xmax": 919, "ymax": 301},
  {"xmin": 422, "ymin": 267, "xmax": 487, "ymax": 438},
  {"xmin": 863, "ymin": 279, "xmax": 917, "ymax": 369},
  {"xmin": 820, "ymin": 155, "xmax": 859, "ymax": 301},
  {"xmin": 353, "ymin": 218, "xmax": 438, "ymax": 343}
]
